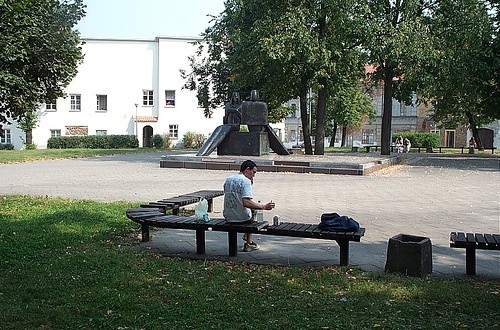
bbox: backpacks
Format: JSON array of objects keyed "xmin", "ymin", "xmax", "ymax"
[{"xmin": 318, "ymin": 212, "xmax": 360, "ymax": 233}]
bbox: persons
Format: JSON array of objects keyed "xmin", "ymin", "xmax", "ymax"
[
  {"xmin": 395, "ymin": 137, "xmax": 403, "ymax": 154},
  {"xmin": 404, "ymin": 138, "xmax": 411, "ymax": 153},
  {"xmin": 469, "ymin": 136, "xmax": 476, "ymax": 148},
  {"xmin": 222, "ymin": 160, "xmax": 275, "ymax": 252}
]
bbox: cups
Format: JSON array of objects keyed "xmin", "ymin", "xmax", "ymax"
[{"xmin": 273, "ymin": 215, "xmax": 280, "ymax": 225}]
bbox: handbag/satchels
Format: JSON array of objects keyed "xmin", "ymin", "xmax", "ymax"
[{"xmin": 195, "ymin": 196, "xmax": 210, "ymax": 224}]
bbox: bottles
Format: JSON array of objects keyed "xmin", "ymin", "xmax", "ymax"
[{"xmin": 256, "ymin": 200, "xmax": 263, "ymax": 224}]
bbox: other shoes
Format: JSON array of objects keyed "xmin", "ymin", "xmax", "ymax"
[{"xmin": 244, "ymin": 240, "xmax": 261, "ymax": 252}]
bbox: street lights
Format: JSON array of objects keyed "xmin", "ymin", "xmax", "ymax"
[
  {"xmin": 135, "ymin": 104, "xmax": 139, "ymax": 138},
  {"xmin": 297, "ymin": 126, "xmax": 301, "ymax": 148}
]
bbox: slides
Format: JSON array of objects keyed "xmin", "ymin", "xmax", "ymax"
[
  {"xmin": 265, "ymin": 125, "xmax": 290, "ymax": 155},
  {"xmin": 196, "ymin": 124, "xmax": 234, "ymax": 156}
]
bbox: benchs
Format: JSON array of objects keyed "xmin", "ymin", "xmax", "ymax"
[
  {"xmin": 437, "ymin": 147, "xmax": 464, "ymax": 153},
  {"xmin": 126, "ymin": 190, "xmax": 365, "ymax": 266},
  {"xmin": 450, "ymin": 232, "xmax": 500, "ymax": 275},
  {"xmin": 364, "ymin": 145, "xmax": 427, "ymax": 153}
]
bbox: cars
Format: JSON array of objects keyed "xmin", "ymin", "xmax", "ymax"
[{"xmin": 292, "ymin": 142, "xmax": 304, "ymax": 148}]
